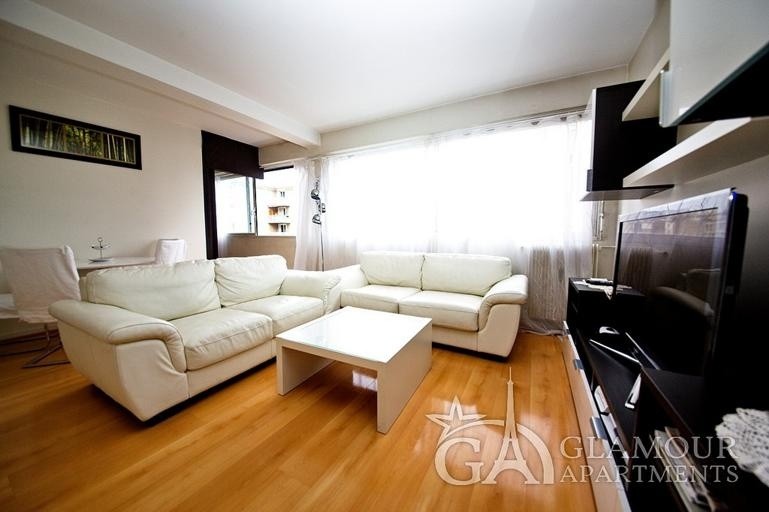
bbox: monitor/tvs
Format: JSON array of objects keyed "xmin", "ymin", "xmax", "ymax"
[{"xmin": 588, "ymin": 186, "xmax": 748, "ymax": 410}]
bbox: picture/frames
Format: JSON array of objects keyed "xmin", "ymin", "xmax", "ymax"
[{"xmin": 9, "ymin": 105, "xmax": 142, "ymax": 171}]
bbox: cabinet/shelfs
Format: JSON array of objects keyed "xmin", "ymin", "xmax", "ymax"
[{"xmin": 563, "ymin": 0, "xmax": 769, "ymax": 512}]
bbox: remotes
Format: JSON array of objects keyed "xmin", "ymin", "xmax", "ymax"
[{"xmin": 586, "ymin": 279, "xmax": 613, "ymax": 286}]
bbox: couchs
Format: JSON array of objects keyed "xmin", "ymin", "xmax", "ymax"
[
  {"xmin": 325, "ymin": 251, "xmax": 529, "ymax": 359},
  {"xmin": 48, "ymin": 255, "xmax": 340, "ymax": 423}
]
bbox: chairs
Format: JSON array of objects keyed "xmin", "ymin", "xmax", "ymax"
[
  {"xmin": 0, "ymin": 246, "xmax": 81, "ymax": 369},
  {"xmin": 155, "ymin": 237, "xmax": 187, "ymax": 266}
]
